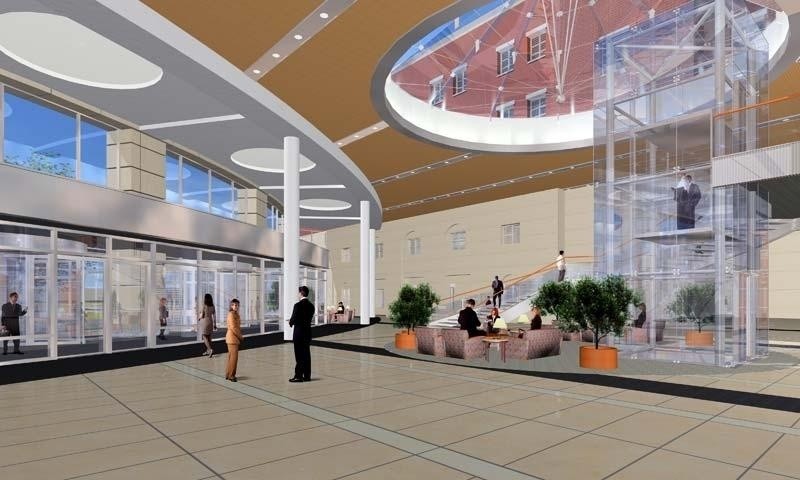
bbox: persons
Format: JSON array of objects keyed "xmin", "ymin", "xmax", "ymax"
[
  {"xmin": 226, "ymin": 299, "xmax": 244, "ymax": 382},
  {"xmin": 288, "ymin": 286, "xmax": 315, "ymax": 382},
  {"xmin": 556, "ymin": 250, "xmax": 566, "ymax": 282},
  {"xmin": 1, "ymin": 292, "xmax": 28, "ymax": 355},
  {"xmin": 518, "ymin": 307, "xmax": 543, "ymax": 339},
  {"xmin": 628, "ymin": 303, "xmax": 646, "ymax": 328},
  {"xmin": 334, "ymin": 302, "xmax": 344, "ymax": 320},
  {"xmin": 197, "ymin": 293, "xmax": 217, "ymax": 358},
  {"xmin": 671, "ymin": 175, "xmax": 702, "ymax": 229},
  {"xmin": 159, "ymin": 297, "xmax": 169, "ymax": 340},
  {"xmin": 458, "ymin": 275, "xmax": 503, "ymax": 338}
]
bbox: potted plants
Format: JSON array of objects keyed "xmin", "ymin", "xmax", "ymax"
[
  {"xmin": 527, "ymin": 271, "xmax": 645, "ymax": 369},
  {"xmin": 388, "ymin": 281, "xmax": 441, "ymax": 350}
]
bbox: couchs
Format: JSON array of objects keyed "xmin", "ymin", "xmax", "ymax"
[{"xmin": 416, "ymin": 324, "xmax": 593, "ymax": 360}]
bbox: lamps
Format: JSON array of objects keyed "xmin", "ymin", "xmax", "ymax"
[
  {"xmin": 492, "ymin": 318, "xmax": 507, "ymax": 339},
  {"xmin": 663, "ymin": 282, "xmax": 728, "ymax": 347},
  {"xmin": 517, "ymin": 314, "xmax": 529, "ymax": 331}
]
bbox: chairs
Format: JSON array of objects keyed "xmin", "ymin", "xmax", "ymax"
[
  {"xmin": 331, "ymin": 307, "xmax": 355, "ymax": 323},
  {"xmin": 646, "ymin": 319, "xmax": 665, "ymax": 342}
]
